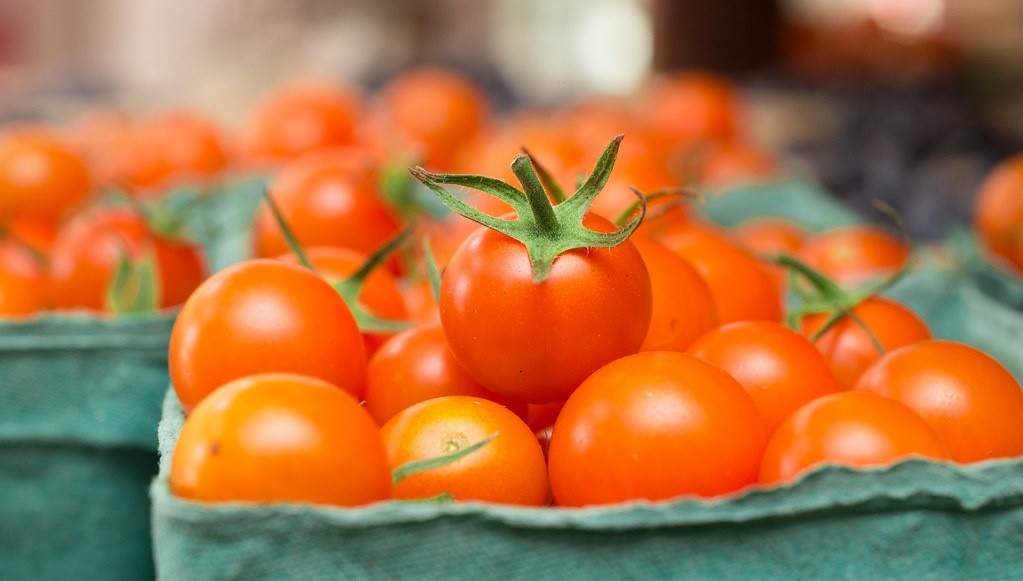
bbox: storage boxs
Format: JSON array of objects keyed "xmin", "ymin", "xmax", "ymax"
[{"xmin": 0, "ymin": 168, "xmax": 1023, "ymax": 580}]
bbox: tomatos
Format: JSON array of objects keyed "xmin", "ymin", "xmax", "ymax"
[{"xmin": 0, "ymin": 66, "xmax": 1023, "ymax": 508}]
